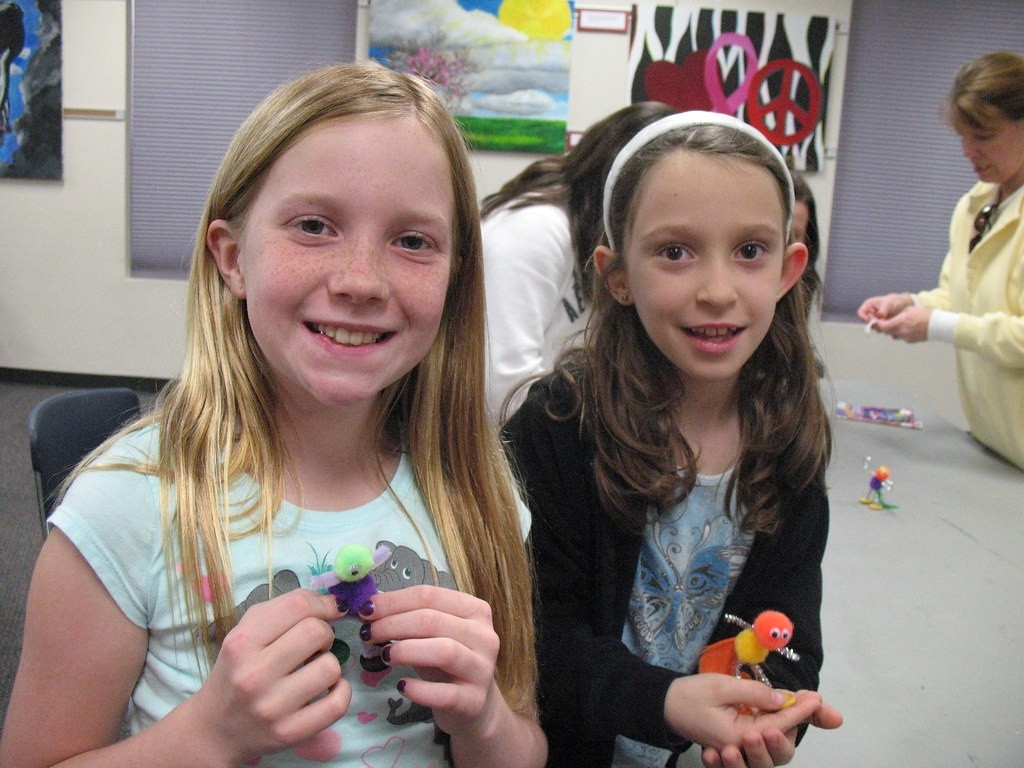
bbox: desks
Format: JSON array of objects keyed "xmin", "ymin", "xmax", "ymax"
[{"xmin": 677, "ymin": 322, "xmax": 1024, "ymax": 768}]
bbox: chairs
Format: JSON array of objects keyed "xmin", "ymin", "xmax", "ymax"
[{"xmin": 29, "ymin": 385, "xmax": 141, "ymax": 540}]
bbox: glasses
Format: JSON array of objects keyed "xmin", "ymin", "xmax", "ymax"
[{"xmin": 969, "ymin": 204, "xmax": 998, "ymax": 252}]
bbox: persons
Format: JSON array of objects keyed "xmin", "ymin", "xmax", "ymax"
[
  {"xmin": 855, "ymin": 50, "xmax": 1024, "ymax": 473},
  {"xmin": 489, "ymin": 111, "xmax": 843, "ymax": 767},
  {"xmin": 1, "ymin": 62, "xmax": 550, "ymax": 767},
  {"xmin": 478, "ymin": 99, "xmax": 674, "ymax": 434}
]
